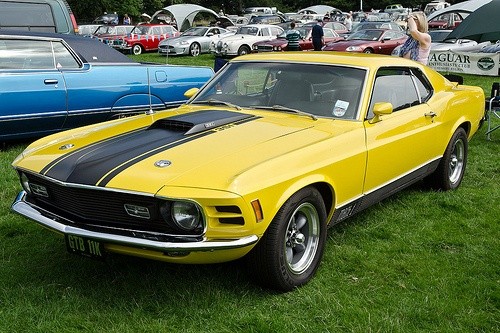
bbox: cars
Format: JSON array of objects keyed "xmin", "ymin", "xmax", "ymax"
[
  {"xmin": 0, "ymin": 35, "xmax": 223, "ymax": 141},
  {"xmin": 93, "ymin": 0, "xmax": 500, "ymax": 57},
  {"xmin": 11, "ymin": 51, "xmax": 487, "ymax": 293}
]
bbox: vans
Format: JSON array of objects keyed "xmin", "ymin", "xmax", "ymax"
[{"xmin": 0, "ymin": 0, "xmax": 79, "ymax": 35}]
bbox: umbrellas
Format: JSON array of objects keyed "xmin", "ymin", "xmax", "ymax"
[{"xmin": 444, "ymin": 0, "xmax": 500, "ymax": 44}]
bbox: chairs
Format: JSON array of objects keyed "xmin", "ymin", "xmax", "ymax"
[
  {"xmin": 214, "ymin": 57, "xmax": 240, "ymax": 95},
  {"xmin": 266, "ymin": 80, "xmax": 313, "ymax": 107},
  {"xmin": 443, "ymin": 74, "xmax": 464, "ymax": 85},
  {"xmin": 483, "ymin": 81, "xmax": 500, "ymax": 141}
]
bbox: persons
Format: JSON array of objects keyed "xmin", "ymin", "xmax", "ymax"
[
  {"xmin": 344, "ymin": 11, "xmax": 353, "ymax": 30},
  {"xmin": 104, "ymin": 13, "xmax": 108, "ymax": 24},
  {"xmin": 312, "ymin": 19, "xmax": 325, "ymax": 51},
  {"xmin": 400, "ymin": 10, "xmax": 431, "ymax": 65},
  {"xmin": 219, "ymin": 10, "xmax": 223, "ymax": 16},
  {"xmin": 286, "ymin": 23, "xmax": 302, "ymax": 51},
  {"xmin": 361, "ymin": 16, "xmax": 367, "ymax": 23},
  {"xmin": 111, "ymin": 12, "xmax": 119, "ymax": 25},
  {"xmin": 124, "ymin": 14, "xmax": 130, "ymax": 25}
]
